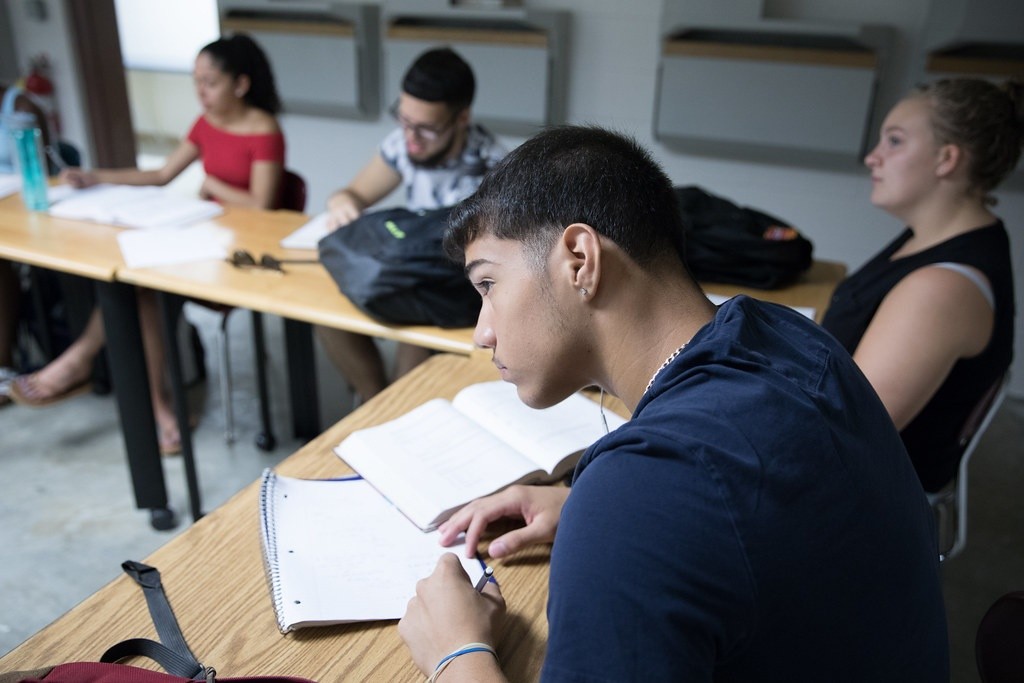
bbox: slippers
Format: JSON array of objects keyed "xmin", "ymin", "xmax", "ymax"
[
  {"xmin": 11, "ymin": 363, "xmax": 91, "ymax": 406},
  {"xmin": 156, "ymin": 408, "xmax": 181, "ymax": 454}
]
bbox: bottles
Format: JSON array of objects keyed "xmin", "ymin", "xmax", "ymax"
[{"xmin": 9, "ymin": 111, "xmax": 50, "ymax": 211}]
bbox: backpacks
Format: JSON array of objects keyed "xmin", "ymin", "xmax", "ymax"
[
  {"xmin": 671, "ymin": 185, "xmax": 813, "ymax": 290},
  {"xmin": 318, "ymin": 203, "xmax": 484, "ymax": 328}
]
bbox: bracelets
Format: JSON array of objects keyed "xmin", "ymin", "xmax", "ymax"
[{"xmin": 427, "ymin": 643, "xmax": 500, "ymax": 683}]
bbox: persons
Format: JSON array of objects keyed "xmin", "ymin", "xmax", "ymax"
[
  {"xmin": 326, "ymin": 47, "xmax": 508, "ymax": 407},
  {"xmin": 399, "ymin": 126, "xmax": 951, "ymax": 683},
  {"xmin": 4, "ymin": 32, "xmax": 287, "ymax": 453},
  {"xmin": 821, "ymin": 78, "xmax": 1024, "ymax": 494}
]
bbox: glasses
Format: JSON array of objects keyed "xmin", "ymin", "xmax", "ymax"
[
  {"xmin": 226, "ymin": 251, "xmax": 284, "ymax": 274},
  {"xmin": 388, "ymin": 98, "xmax": 463, "ymax": 143}
]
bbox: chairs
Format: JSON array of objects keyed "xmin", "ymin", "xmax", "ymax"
[
  {"xmin": 180, "ymin": 169, "xmax": 308, "ymax": 451},
  {"xmin": 935, "ymin": 365, "xmax": 1013, "ymax": 589}
]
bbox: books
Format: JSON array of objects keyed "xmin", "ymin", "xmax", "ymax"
[
  {"xmin": 50, "ymin": 180, "xmax": 233, "ymax": 270},
  {"xmin": 281, "ymin": 211, "xmax": 330, "ymax": 249},
  {"xmin": 334, "ymin": 379, "xmax": 630, "ymax": 533},
  {"xmin": 260, "ymin": 466, "xmax": 500, "ymax": 635}
]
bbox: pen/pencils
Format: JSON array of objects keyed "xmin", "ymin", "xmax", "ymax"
[{"xmin": 475, "ymin": 567, "xmax": 493, "ymax": 591}]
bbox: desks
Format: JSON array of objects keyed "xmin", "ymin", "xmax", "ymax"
[{"xmin": 0, "ymin": 171, "xmax": 848, "ymax": 683}]
